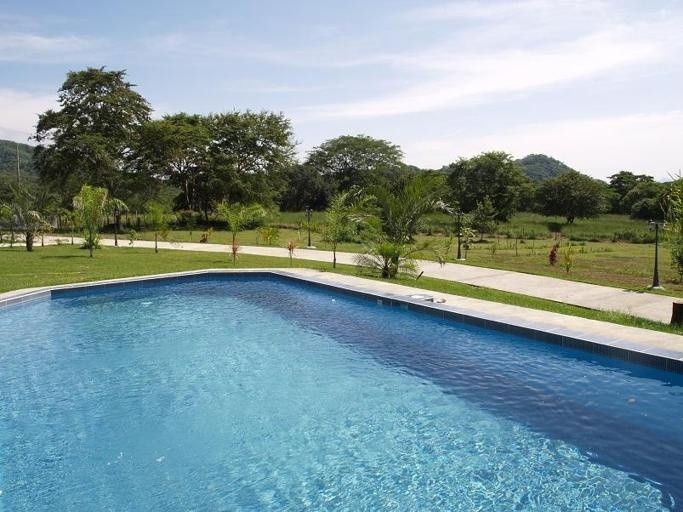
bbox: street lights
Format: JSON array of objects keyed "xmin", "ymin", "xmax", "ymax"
[
  {"xmin": 111, "ymin": 203, "xmax": 121, "ymax": 245},
  {"xmin": 304, "ymin": 204, "xmax": 314, "ymax": 247},
  {"xmin": 455, "ymin": 208, "xmax": 465, "ymax": 261},
  {"xmin": 647, "ymin": 219, "xmax": 671, "ymax": 292}
]
